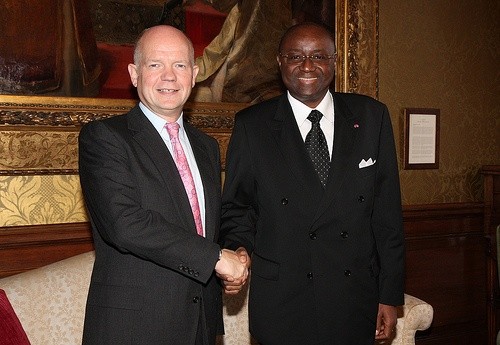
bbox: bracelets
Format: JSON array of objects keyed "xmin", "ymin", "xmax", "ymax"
[{"xmin": 218, "ymin": 251, "xmax": 222, "ymax": 260}]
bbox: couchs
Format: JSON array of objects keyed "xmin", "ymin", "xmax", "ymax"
[{"xmin": 0, "ymin": 249, "xmax": 433, "ymax": 345}]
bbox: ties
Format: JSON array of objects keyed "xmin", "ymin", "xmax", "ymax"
[
  {"xmin": 305, "ymin": 110, "xmax": 331, "ymax": 193},
  {"xmin": 164, "ymin": 122, "xmax": 203, "ymax": 236}
]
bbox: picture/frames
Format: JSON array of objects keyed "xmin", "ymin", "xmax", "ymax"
[{"xmin": 0, "ymin": 0, "xmax": 379, "ymax": 176}]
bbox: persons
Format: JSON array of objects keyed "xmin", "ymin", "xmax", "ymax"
[
  {"xmin": 78, "ymin": 24, "xmax": 249, "ymax": 345},
  {"xmin": 219, "ymin": 21, "xmax": 403, "ymax": 345}
]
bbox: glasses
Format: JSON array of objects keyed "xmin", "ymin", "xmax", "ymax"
[{"xmin": 279, "ymin": 52, "xmax": 334, "ymax": 65}]
